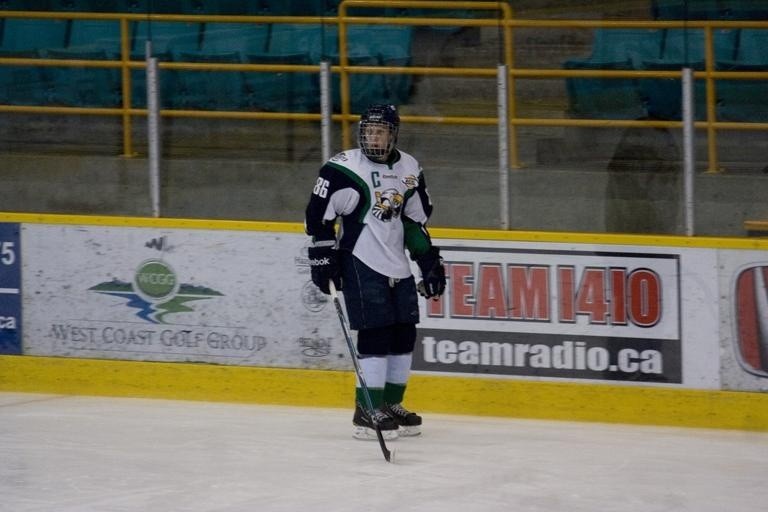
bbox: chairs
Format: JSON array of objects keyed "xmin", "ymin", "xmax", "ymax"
[{"xmin": 558, "ymin": 0, "xmax": 767, "ymax": 129}]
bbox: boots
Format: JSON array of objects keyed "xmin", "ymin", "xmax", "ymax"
[
  {"xmin": 386, "ymin": 400, "xmax": 423, "ymax": 435},
  {"xmin": 352, "ymin": 402, "xmax": 399, "ymax": 443}
]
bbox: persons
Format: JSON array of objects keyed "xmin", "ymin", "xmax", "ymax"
[{"xmin": 301, "ymin": 102, "xmax": 447, "ymax": 433}]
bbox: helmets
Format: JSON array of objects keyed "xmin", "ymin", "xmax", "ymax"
[{"xmin": 357, "ymin": 103, "xmax": 398, "ymax": 159}]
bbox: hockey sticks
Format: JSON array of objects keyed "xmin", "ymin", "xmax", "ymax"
[{"xmin": 329, "ymin": 281, "xmax": 401, "ymax": 461}]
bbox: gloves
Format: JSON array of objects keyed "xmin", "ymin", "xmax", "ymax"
[
  {"xmin": 416, "ymin": 254, "xmax": 451, "ymax": 302},
  {"xmin": 308, "ymin": 245, "xmax": 346, "ymax": 296}
]
